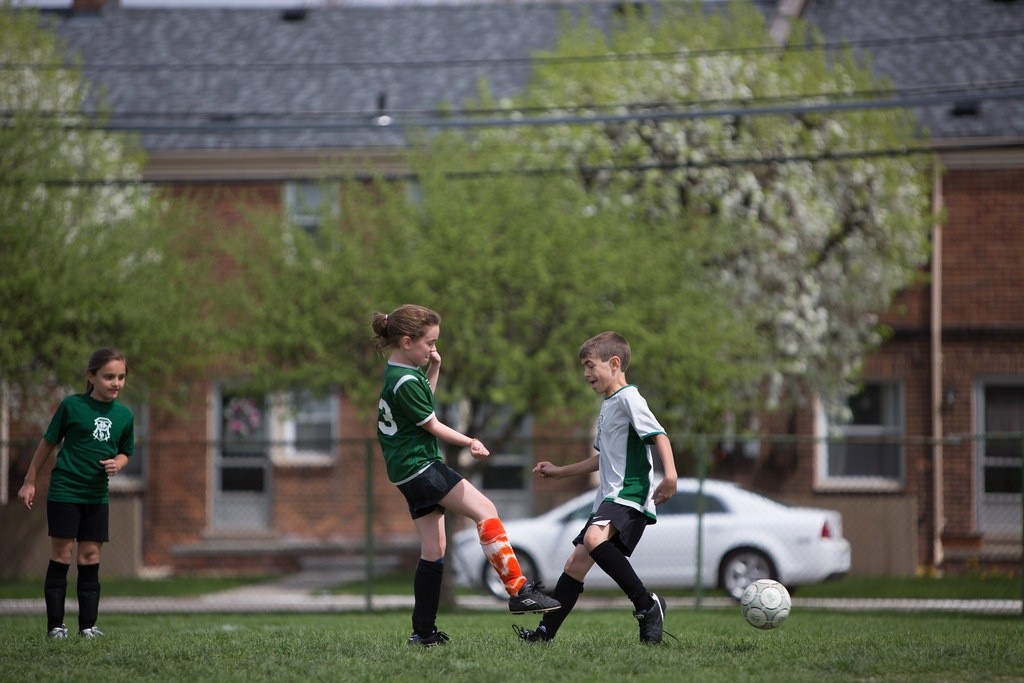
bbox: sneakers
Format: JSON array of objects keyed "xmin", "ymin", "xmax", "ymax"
[
  {"xmin": 78, "ymin": 626, "xmax": 103, "ymax": 640},
  {"xmin": 511, "ymin": 624, "xmax": 553, "ymax": 647},
  {"xmin": 633, "ymin": 592, "xmax": 666, "ymax": 646},
  {"xmin": 509, "ymin": 580, "xmax": 561, "ymax": 615},
  {"xmin": 406, "ymin": 631, "xmax": 450, "ymax": 649},
  {"xmin": 47, "ymin": 624, "xmax": 68, "ymax": 640}
]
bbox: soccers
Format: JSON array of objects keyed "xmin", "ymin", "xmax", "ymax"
[{"xmin": 741, "ymin": 578, "xmax": 791, "ymax": 630}]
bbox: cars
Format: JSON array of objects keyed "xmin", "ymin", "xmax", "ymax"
[{"xmin": 449, "ymin": 475, "xmax": 852, "ymax": 602}]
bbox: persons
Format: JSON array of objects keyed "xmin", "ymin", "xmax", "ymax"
[
  {"xmin": 18, "ymin": 348, "xmax": 135, "ymax": 638},
  {"xmin": 371, "ymin": 305, "xmax": 562, "ymax": 649},
  {"xmin": 517, "ymin": 333, "xmax": 677, "ymax": 644}
]
